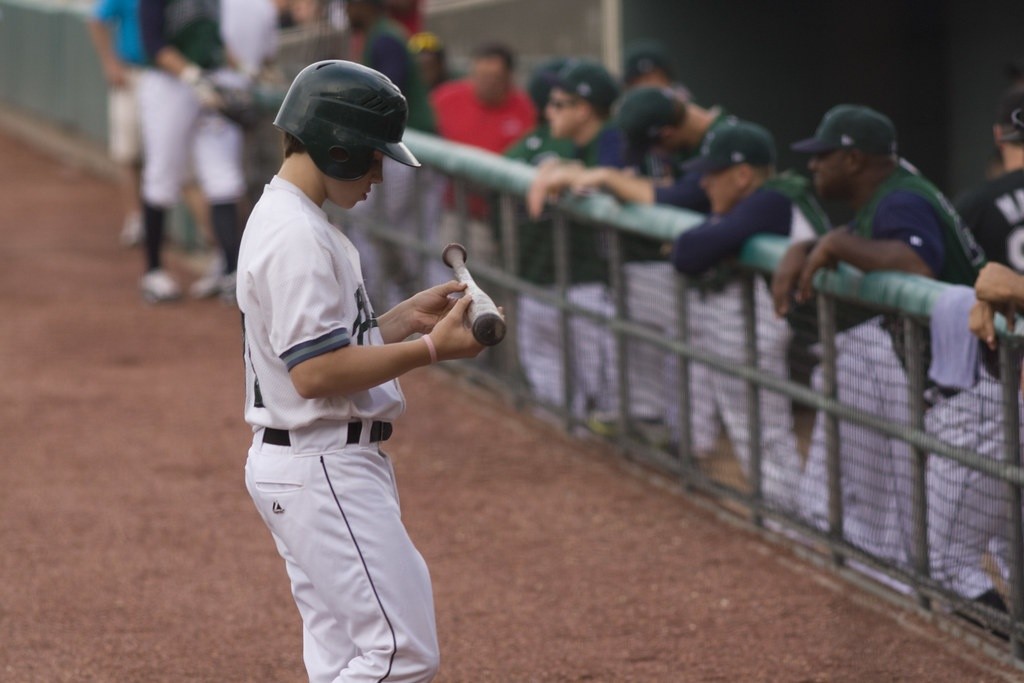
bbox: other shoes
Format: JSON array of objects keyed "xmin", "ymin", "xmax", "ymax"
[
  {"xmin": 140, "ymin": 270, "xmax": 179, "ymax": 299},
  {"xmin": 123, "ymin": 220, "xmax": 145, "ymax": 245},
  {"xmin": 218, "ymin": 275, "xmax": 240, "ymax": 302}
]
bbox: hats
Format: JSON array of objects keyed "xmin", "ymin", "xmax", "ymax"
[
  {"xmin": 536, "ymin": 57, "xmax": 617, "ymax": 110},
  {"xmin": 790, "ymin": 102, "xmax": 897, "ymax": 159},
  {"xmin": 615, "ymin": 85, "xmax": 676, "ymax": 168},
  {"xmin": 677, "ymin": 117, "xmax": 775, "ymax": 175}
]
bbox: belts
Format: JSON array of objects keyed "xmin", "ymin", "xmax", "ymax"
[{"xmin": 265, "ymin": 417, "xmax": 394, "ymax": 446}]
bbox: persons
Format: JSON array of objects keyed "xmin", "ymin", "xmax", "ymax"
[
  {"xmin": 86, "ymin": 0, "xmax": 1024, "ymax": 634},
  {"xmin": 235, "ymin": 59, "xmax": 503, "ymax": 683}
]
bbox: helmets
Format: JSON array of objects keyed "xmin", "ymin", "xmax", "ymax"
[{"xmin": 272, "ymin": 59, "xmax": 422, "ymax": 180}]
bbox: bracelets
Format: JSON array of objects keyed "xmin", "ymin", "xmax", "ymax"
[{"xmin": 423, "ymin": 335, "xmax": 437, "ymax": 363}]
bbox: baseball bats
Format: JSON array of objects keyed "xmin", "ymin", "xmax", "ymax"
[{"xmin": 439, "ymin": 241, "xmax": 507, "ymax": 348}]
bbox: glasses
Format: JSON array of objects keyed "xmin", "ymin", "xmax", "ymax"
[{"xmin": 544, "ymin": 94, "xmax": 579, "ymax": 109}]
